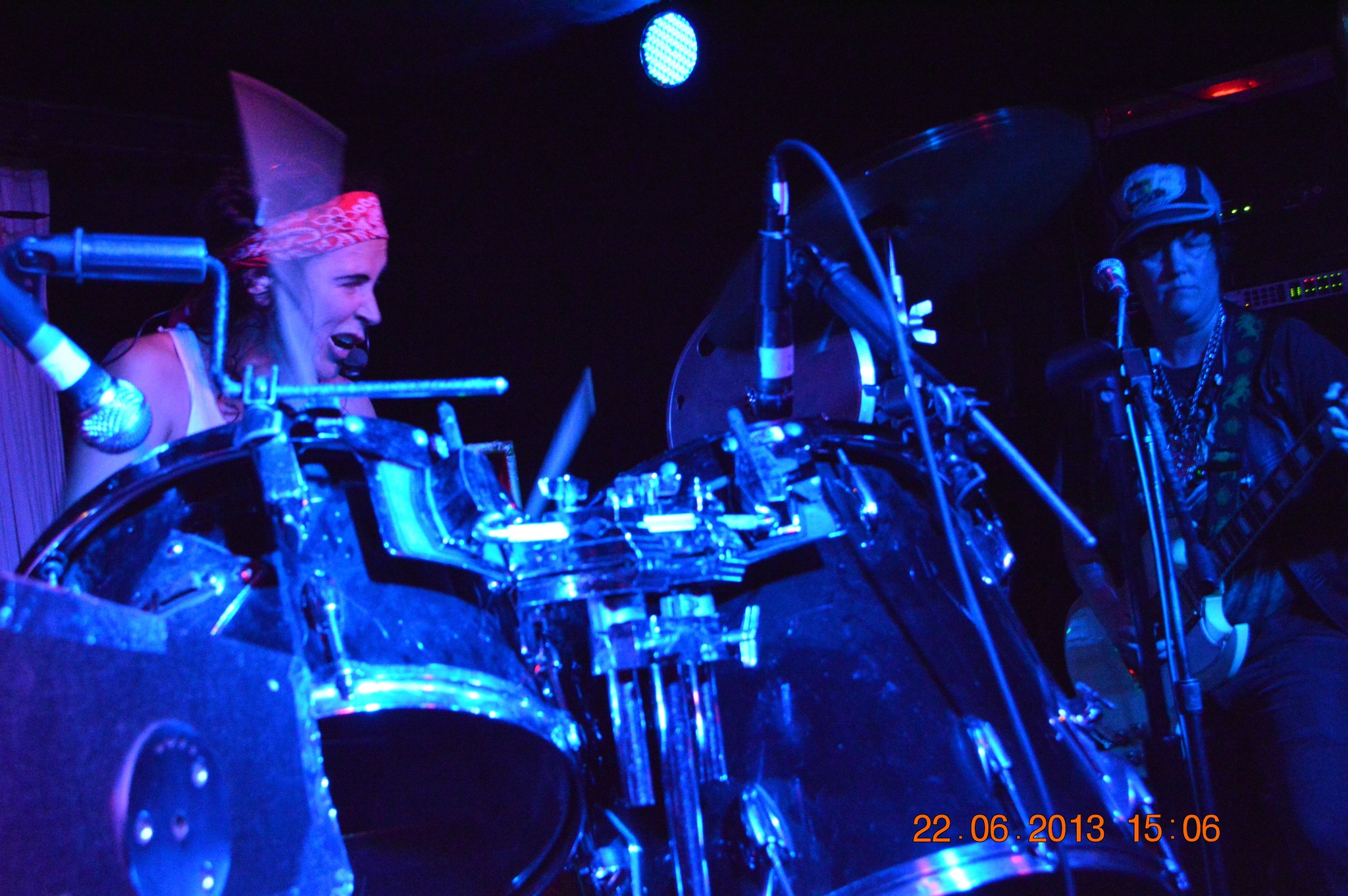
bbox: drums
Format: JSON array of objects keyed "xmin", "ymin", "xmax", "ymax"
[
  {"xmin": 0, "ymin": 399, "xmax": 589, "ymax": 896},
  {"xmin": 612, "ymin": 439, "xmax": 1164, "ymax": 894}
]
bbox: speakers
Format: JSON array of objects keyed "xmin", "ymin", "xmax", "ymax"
[{"xmin": 0, "ymin": 571, "xmax": 359, "ymax": 896}]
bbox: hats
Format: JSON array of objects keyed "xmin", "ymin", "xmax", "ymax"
[
  {"xmin": 206, "ymin": 70, "xmax": 389, "ymax": 270},
  {"xmin": 1113, "ymin": 164, "xmax": 1222, "ymax": 249}
]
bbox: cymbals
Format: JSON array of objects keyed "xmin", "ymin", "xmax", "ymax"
[{"xmin": 663, "ymin": 101, "xmax": 1100, "ymax": 453}]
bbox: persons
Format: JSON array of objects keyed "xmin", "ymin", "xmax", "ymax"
[
  {"xmin": 1051, "ymin": 165, "xmax": 1348, "ymax": 896},
  {"xmin": 62, "ymin": 68, "xmax": 389, "ymax": 506}
]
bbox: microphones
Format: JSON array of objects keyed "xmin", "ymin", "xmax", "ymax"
[
  {"xmin": 0, "ymin": 271, "xmax": 149, "ymax": 454},
  {"xmin": 1091, "ymin": 259, "xmax": 1130, "ymax": 301},
  {"xmin": 756, "ymin": 151, "xmax": 796, "ymax": 422}
]
